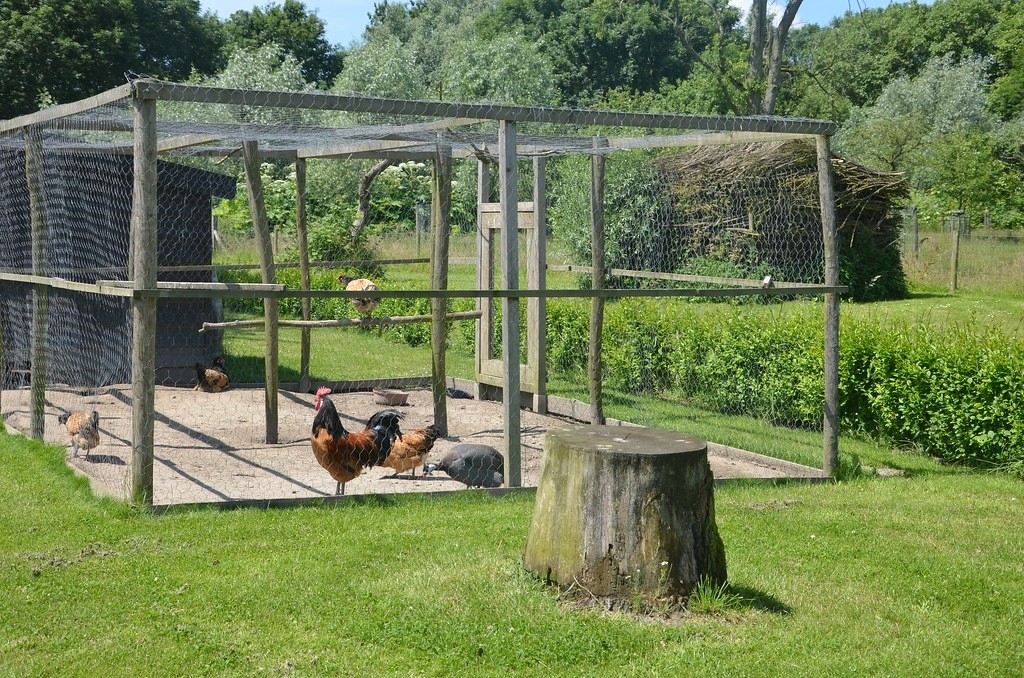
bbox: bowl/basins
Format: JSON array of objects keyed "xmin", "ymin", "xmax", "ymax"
[{"xmin": 372, "ymin": 388, "xmax": 408, "ymax": 405}]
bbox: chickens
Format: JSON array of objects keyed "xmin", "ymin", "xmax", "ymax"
[
  {"xmin": 194, "ymin": 356, "xmax": 229, "ymax": 393},
  {"xmin": 58, "ymin": 410, "xmax": 100, "ymax": 461},
  {"xmin": 338, "ymin": 274, "xmax": 381, "ymax": 321},
  {"xmin": 311, "ymin": 385, "xmax": 505, "ymax": 494}
]
